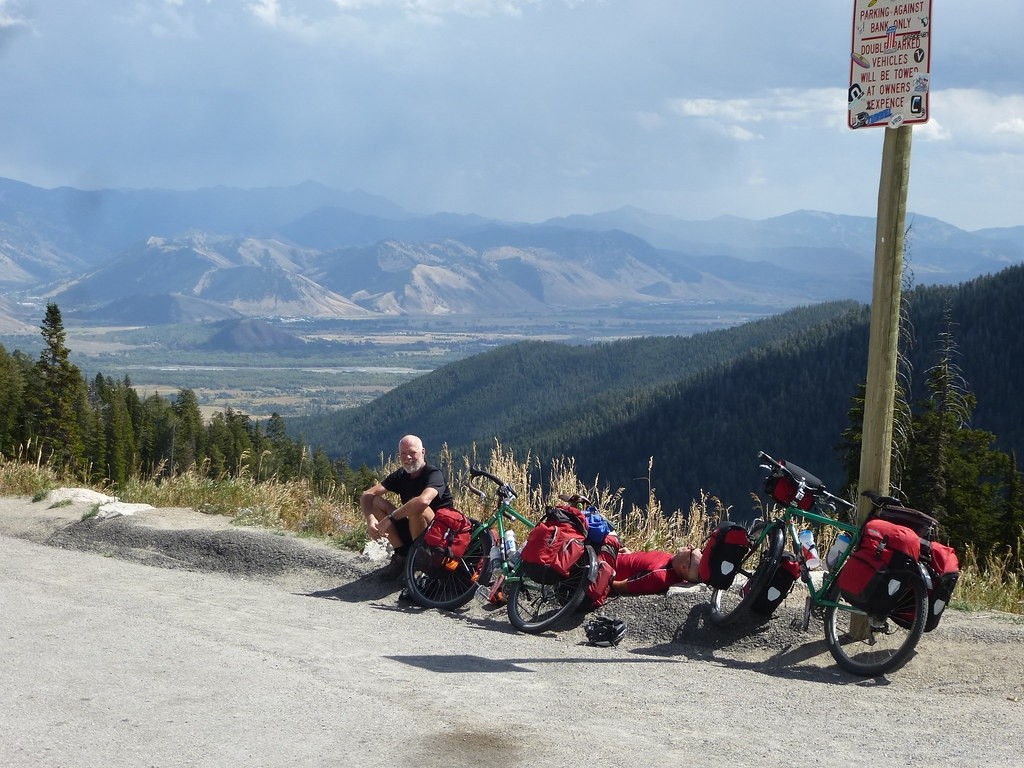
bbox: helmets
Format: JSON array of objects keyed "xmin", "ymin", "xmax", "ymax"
[{"xmin": 586, "ymin": 616, "xmax": 628, "ymax": 648}]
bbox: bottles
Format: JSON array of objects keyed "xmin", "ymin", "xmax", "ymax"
[
  {"xmin": 504, "ymin": 529, "xmax": 516, "ymax": 554},
  {"xmin": 509, "ymin": 540, "xmax": 527, "ymax": 568},
  {"xmin": 490, "ymin": 545, "xmax": 501, "ymax": 571},
  {"xmin": 822, "ymin": 536, "xmax": 850, "ymax": 570},
  {"xmin": 799, "ymin": 530, "xmax": 819, "ymax": 568}
]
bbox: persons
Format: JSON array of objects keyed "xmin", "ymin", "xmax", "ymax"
[
  {"xmin": 358, "ymin": 432, "xmax": 460, "ymax": 581},
  {"xmin": 611, "ymin": 543, "xmax": 704, "ymax": 594}
]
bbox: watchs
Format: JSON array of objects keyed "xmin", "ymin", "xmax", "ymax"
[{"xmin": 388, "ymin": 512, "xmax": 398, "ymax": 522}]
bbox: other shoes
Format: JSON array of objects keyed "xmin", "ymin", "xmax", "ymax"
[
  {"xmin": 377, "ymin": 553, "xmax": 409, "ymax": 583},
  {"xmin": 398, "ymin": 573, "xmax": 439, "ymax": 601}
]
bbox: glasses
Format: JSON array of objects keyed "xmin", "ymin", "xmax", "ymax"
[{"xmin": 686, "ymin": 547, "xmax": 701, "ymax": 570}]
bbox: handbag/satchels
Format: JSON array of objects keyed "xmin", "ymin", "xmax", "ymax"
[
  {"xmin": 743, "ymin": 550, "xmax": 800, "ymax": 617},
  {"xmin": 582, "ymin": 505, "xmax": 610, "ymax": 543},
  {"xmin": 698, "ymin": 521, "xmax": 751, "ymax": 592},
  {"xmin": 834, "ymin": 519, "xmax": 921, "ymax": 622},
  {"xmin": 522, "ymin": 504, "xmax": 589, "ymax": 585},
  {"xmin": 881, "ymin": 504, "xmax": 941, "ymax": 558},
  {"xmin": 553, "ymin": 535, "xmax": 620, "ymax": 609},
  {"xmin": 422, "ymin": 509, "xmax": 472, "ymax": 559},
  {"xmin": 765, "ymin": 459, "xmax": 822, "ymax": 512},
  {"xmin": 890, "ymin": 542, "xmax": 960, "ymax": 633}
]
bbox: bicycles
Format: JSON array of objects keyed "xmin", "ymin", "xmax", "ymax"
[
  {"xmin": 404, "ymin": 465, "xmax": 599, "ymax": 635},
  {"xmin": 708, "ymin": 450, "xmax": 937, "ymax": 676}
]
